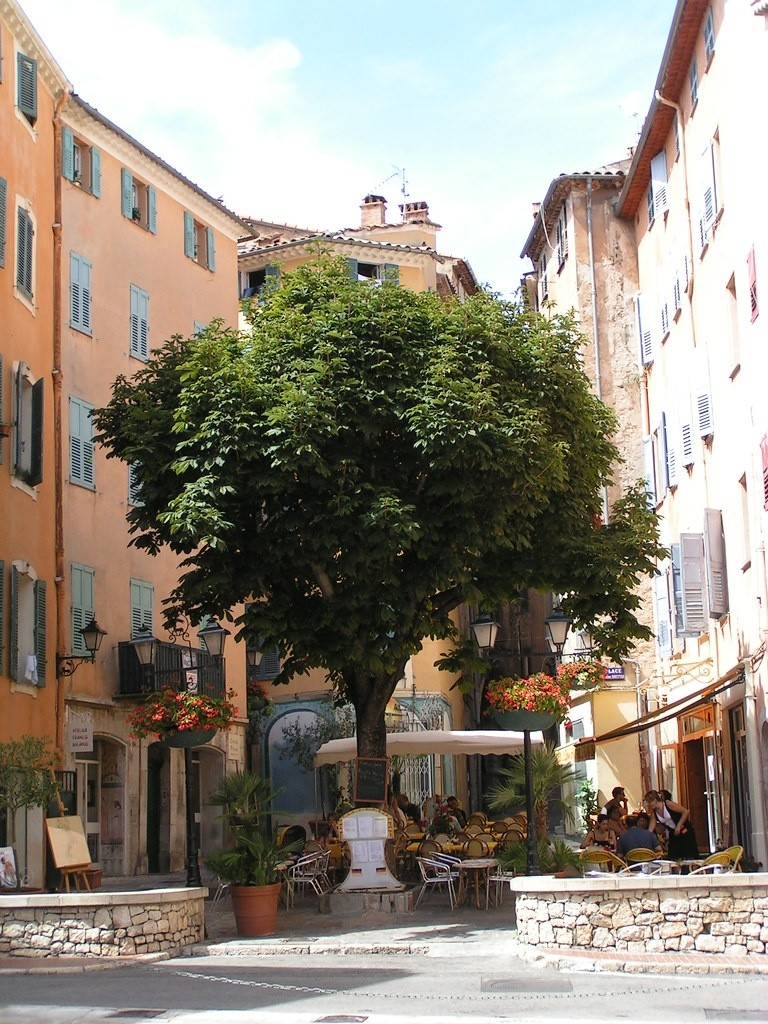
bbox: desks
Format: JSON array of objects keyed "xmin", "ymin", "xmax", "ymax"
[
  {"xmin": 452, "ymin": 859, "xmax": 499, "ymax": 910},
  {"xmin": 407, "ymin": 842, "xmax": 501, "ymax": 855},
  {"xmin": 409, "ymin": 832, "xmax": 527, "ymax": 840}
]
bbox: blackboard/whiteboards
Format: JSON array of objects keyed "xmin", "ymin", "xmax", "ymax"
[{"xmin": 353, "ymin": 758, "xmax": 389, "ymax": 803}]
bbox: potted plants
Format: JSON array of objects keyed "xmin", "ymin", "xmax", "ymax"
[
  {"xmin": 203, "ymin": 768, "xmax": 305, "ymax": 937},
  {"xmin": 483, "ymin": 738, "xmax": 591, "ymax": 878}
]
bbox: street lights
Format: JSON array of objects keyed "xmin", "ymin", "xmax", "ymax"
[
  {"xmin": 471, "ymin": 607, "xmax": 571, "ymax": 875},
  {"xmin": 128, "ymin": 614, "xmax": 231, "ymax": 887}
]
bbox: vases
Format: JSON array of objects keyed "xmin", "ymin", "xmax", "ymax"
[
  {"xmin": 249, "ymin": 697, "xmax": 268, "ymax": 710},
  {"xmin": 79, "ymin": 870, "xmax": 104, "ymax": 889},
  {"xmin": 492, "ymin": 709, "xmax": 561, "ymax": 729},
  {"xmin": 162, "ymin": 728, "xmax": 217, "ymax": 749},
  {"xmin": 571, "ymin": 680, "xmax": 599, "ymax": 691}
]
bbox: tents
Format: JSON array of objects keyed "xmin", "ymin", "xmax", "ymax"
[{"xmin": 312, "ymin": 730, "xmax": 544, "ymax": 840}]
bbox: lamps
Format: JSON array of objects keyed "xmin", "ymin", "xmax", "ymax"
[{"xmin": 56, "ymin": 618, "xmax": 108, "ymax": 680}]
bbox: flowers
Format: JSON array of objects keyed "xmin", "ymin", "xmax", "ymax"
[
  {"xmin": 557, "ymin": 659, "xmax": 609, "ymax": 685},
  {"xmin": 247, "ymin": 679, "xmax": 273, "ymax": 717},
  {"xmin": 481, "ymin": 671, "xmax": 573, "ymax": 729},
  {"xmin": 126, "ymin": 674, "xmax": 239, "ymax": 740}
]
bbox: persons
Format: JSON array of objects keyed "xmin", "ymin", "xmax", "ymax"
[
  {"xmin": 447, "ymin": 796, "xmax": 466, "ymax": 828},
  {"xmin": 645, "ymin": 790, "xmax": 699, "ymax": 860},
  {"xmin": 581, "ymin": 787, "xmax": 671, "ymax": 871},
  {"xmin": 399, "ymin": 794, "xmax": 421, "ymax": 822}
]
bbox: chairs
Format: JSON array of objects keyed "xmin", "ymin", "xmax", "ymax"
[
  {"xmin": 579, "ymin": 845, "xmax": 745, "ymax": 874},
  {"xmin": 208, "ymin": 809, "xmax": 529, "ymax": 912}
]
